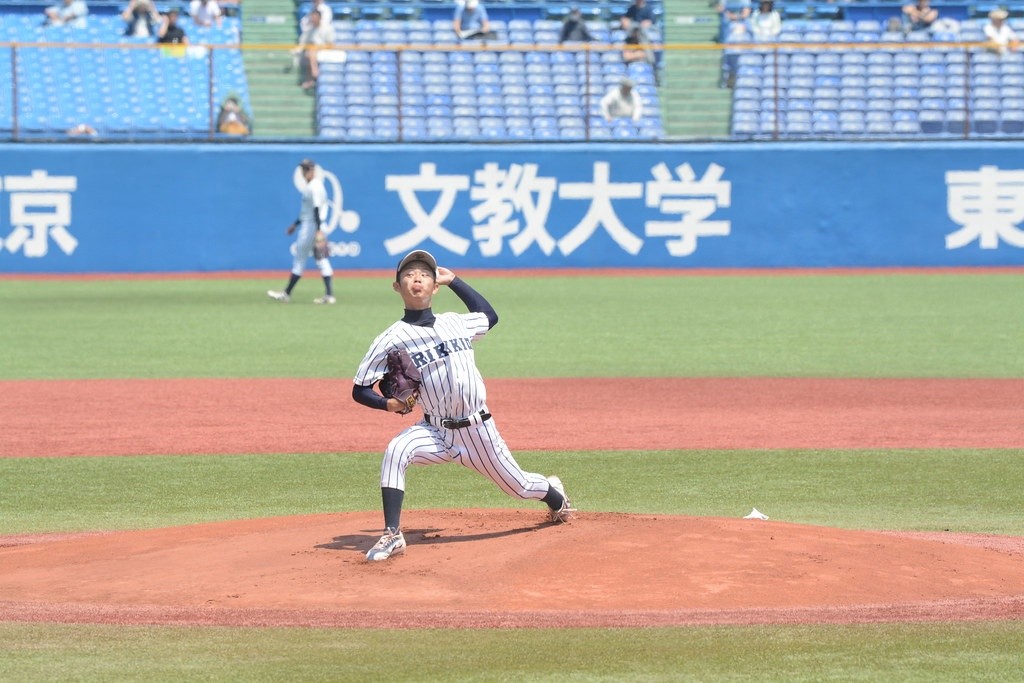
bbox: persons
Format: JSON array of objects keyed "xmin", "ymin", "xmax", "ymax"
[
  {"xmin": 292, "ymin": 0, "xmax": 336, "ymax": 90},
  {"xmin": 901, "ymin": 0, "xmax": 961, "ymax": 35},
  {"xmin": 619, "ymin": 0, "xmax": 658, "ymax": 63},
  {"xmin": 217, "ymin": 96, "xmax": 252, "ymax": 136},
  {"xmin": 352, "ymin": 250, "xmax": 578, "ymax": 562},
  {"xmin": 715, "ymin": 0, "xmax": 782, "ymax": 40},
  {"xmin": 122, "ymin": 0, "xmax": 224, "ymax": 45},
  {"xmin": 558, "ymin": 7, "xmax": 598, "ymax": 45},
  {"xmin": 599, "ymin": 79, "xmax": 642, "ymax": 123},
  {"xmin": 267, "ymin": 159, "xmax": 339, "ymax": 305},
  {"xmin": 981, "ymin": 8, "xmax": 1018, "ymax": 54},
  {"xmin": 453, "ymin": 0, "xmax": 490, "ymax": 40},
  {"xmin": 44, "ymin": 0, "xmax": 89, "ymax": 30}
]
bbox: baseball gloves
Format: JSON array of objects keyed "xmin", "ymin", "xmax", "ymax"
[
  {"xmin": 378, "ymin": 348, "xmax": 421, "ymax": 415},
  {"xmin": 314, "ymin": 231, "xmax": 329, "ymax": 260}
]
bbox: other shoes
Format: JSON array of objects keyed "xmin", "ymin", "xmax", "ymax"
[
  {"xmin": 314, "ymin": 295, "xmax": 336, "ymax": 304},
  {"xmin": 268, "ymin": 291, "xmax": 290, "ymax": 302}
]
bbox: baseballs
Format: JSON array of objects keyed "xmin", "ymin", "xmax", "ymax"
[{"xmin": 436, "ymin": 269, "xmax": 439, "ymax": 278}]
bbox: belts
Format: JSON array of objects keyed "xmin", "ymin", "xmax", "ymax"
[{"xmin": 424, "ymin": 409, "xmax": 491, "ymax": 430}]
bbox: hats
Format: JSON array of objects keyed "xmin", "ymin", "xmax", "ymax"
[
  {"xmin": 396, "ymin": 250, "xmax": 439, "ymax": 283},
  {"xmin": 299, "ymin": 159, "xmax": 314, "ymax": 167}
]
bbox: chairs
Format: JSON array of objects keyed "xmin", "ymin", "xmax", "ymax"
[
  {"xmin": 293, "ymin": 0, "xmax": 665, "ymax": 140},
  {"xmin": 0, "ymin": 0, "xmax": 254, "ymax": 133},
  {"xmin": 720, "ymin": 0, "xmax": 1024, "ymax": 138}
]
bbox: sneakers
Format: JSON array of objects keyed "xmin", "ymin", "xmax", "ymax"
[
  {"xmin": 548, "ymin": 476, "xmax": 577, "ymax": 524},
  {"xmin": 366, "ymin": 527, "xmax": 406, "ymax": 561}
]
